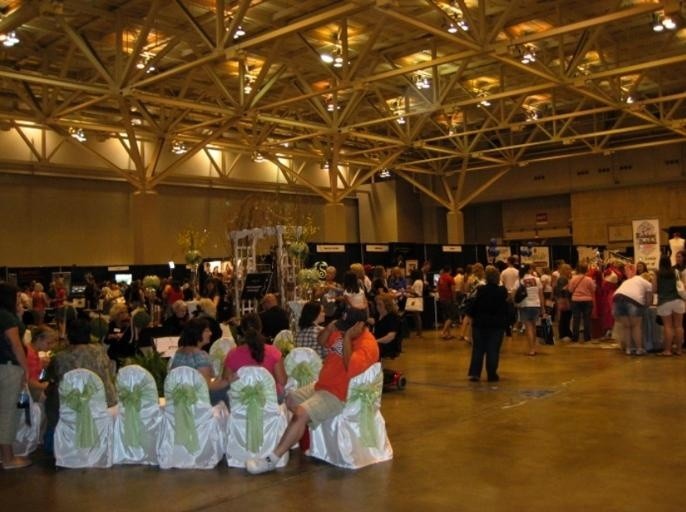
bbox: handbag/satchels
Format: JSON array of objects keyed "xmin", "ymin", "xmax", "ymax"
[
  {"xmin": 404, "ymin": 296, "xmax": 425, "ymax": 313},
  {"xmin": 540, "ymin": 315, "xmax": 555, "ymax": 346},
  {"xmin": 514, "ymin": 284, "xmax": 528, "ymax": 304},
  {"xmin": 675, "ymin": 279, "xmax": 686, "ymax": 301},
  {"xmin": 553, "ymin": 295, "xmax": 572, "ymax": 312}
]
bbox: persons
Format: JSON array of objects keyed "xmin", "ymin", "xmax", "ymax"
[
  {"xmin": 258, "ymin": 293, "xmax": 290, "ymax": 338},
  {"xmin": 55, "ymin": 320, "xmax": 119, "ymax": 408},
  {"xmin": 293, "ymin": 300, "xmax": 327, "ymax": 357},
  {"xmin": 388, "ymin": 258, "xmax": 485, "ymax": 340},
  {"xmin": 0, "ymin": 280, "xmax": 30, "ymax": 469},
  {"xmin": 671, "ymin": 252, "xmax": 686, "ymax": 349},
  {"xmin": 170, "ymin": 319, "xmax": 240, "ymax": 413},
  {"xmin": 17, "ymin": 271, "xmax": 107, "ymax": 348},
  {"xmin": 223, "ymin": 312, "xmax": 288, "ymax": 406},
  {"xmin": 636, "ymin": 263, "xmax": 653, "ymax": 351},
  {"xmin": 541, "ymin": 263, "xmax": 596, "ymax": 342},
  {"xmin": 244, "ymin": 306, "xmax": 380, "ymax": 475},
  {"xmin": 511, "ymin": 264, "xmax": 545, "ymax": 357},
  {"xmin": 315, "ymin": 260, "xmax": 388, "ymax": 327},
  {"xmin": 655, "ymin": 254, "xmax": 685, "ymax": 356},
  {"xmin": 25, "ymin": 326, "xmax": 54, "ymax": 403},
  {"xmin": 368, "ymin": 292, "xmax": 403, "ymax": 360},
  {"xmin": 498, "ymin": 257, "xmax": 521, "ymax": 337},
  {"xmin": 465, "ymin": 264, "xmax": 518, "ymax": 383},
  {"xmin": 107, "ymin": 263, "xmax": 201, "ymax": 372},
  {"xmin": 201, "ymin": 260, "xmax": 237, "ymax": 355},
  {"xmin": 612, "ymin": 271, "xmax": 653, "ymax": 355}
]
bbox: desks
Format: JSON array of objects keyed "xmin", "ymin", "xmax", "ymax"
[{"xmin": 154, "ymin": 334, "xmax": 184, "ymax": 359}]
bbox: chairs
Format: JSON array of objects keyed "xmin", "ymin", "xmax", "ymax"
[
  {"xmin": 273, "ymin": 330, "xmax": 294, "ymax": 353},
  {"xmin": 114, "ymin": 364, "xmax": 162, "ymax": 465},
  {"xmin": 305, "ymin": 362, "xmax": 394, "ymax": 469},
  {"xmin": 159, "ymin": 366, "xmax": 229, "ymax": 469},
  {"xmin": 227, "ymin": 365, "xmax": 290, "ymax": 467},
  {"xmin": 54, "ymin": 369, "xmax": 114, "ymax": 467},
  {"xmin": 208, "ymin": 338, "xmax": 238, "ymax": 377},
  {"xmin": 284, "ymin": 347, "xmax": 323, "ymax": 394}
]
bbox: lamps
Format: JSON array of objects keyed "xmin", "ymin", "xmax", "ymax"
[
  {"xmin": 380, "ymin": 169, "xmax": 389, "ymax": 178},
  {"xmin": 416, "ymin": 76, "xmax": 430, "ymax": 90},
  {"xmin": 448, "ymin": 16, "xmax": 468, "ymax": 33},
  {"xmin": 477, "ymin": 94, "xmax": 491, "ymax": 108},
  {"xmin": 524, "ymin": 109, "xmax": 536, "ymax": 123},
  {"xmin": 1, "ymin": 31, "xmax": 19, "ymax": 47},
  {"xmin": 320, "ymin": 49, "xmax": 343, "ymax": 68},
  {"xmin": 137, "ymin": 57, "xmax": 155, "ymax": 74},
  {"xmin": 132, "ymin": 118, "xmax": 143, "ymax": 127},
  {"xmin": 233, "ymin": 26, "xmax": 245, "ymax": 39},
  {"xmin": 244, "ymin": 80, "xmax": 252, "ymax": 95},
  {"xmin": 249, "ymin": 152, "xmax": 264, "ymax": 164},
  {"xmin": 327, "ymin": 102, "xmax": 339, "ymax": 112},
  {"xmin": 170, "ymin": 139, "xmax": 187, "ymax": 155},
  {"xmin": 520, "ymin": 51, "xmax": 535, "ymax": 64},
  {"xmin": 68, "ymin": 127, "xmax": 86, "ymax": 142},
  {"xmin": 396, "ymin": 117, "xmax": 406, "ymax": 125},
  {"xmin": 652, "ymin": 12, "xmax": 676, "ymax": 31}
]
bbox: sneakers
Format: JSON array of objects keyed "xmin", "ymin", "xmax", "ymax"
[
  {"xmin": 672, "ymin": 351, "xmax": 681, "ymax": 356},
  {"xmin": 656, "ymin": 351, "xmax": 673, "ymax": 356},
  {"xmin": 244, "ymin": 455, "xmax": 276, "ymax": 475},
  {"xmin": 487, "ymin": 373, "xmax": 501, "ymax": 382},
  {"xmin": 3, "ymin": 457, "xmax": 33, "ymax": 470},
  {"xmin": 467, "ymin": 375, "xmax": 481, "ymax": 382},
  {"xmin": 624, "ymin": 348, "xmax": 632, "ymax": 355},
  {"xmin": 635, "ymin": 348, "xmax": 647, "ymax": 356}
]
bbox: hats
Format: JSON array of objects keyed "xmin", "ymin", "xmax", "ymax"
[{"xmin": 334, "ymin": 308, "xmax": 369, "ymax": 332}]
